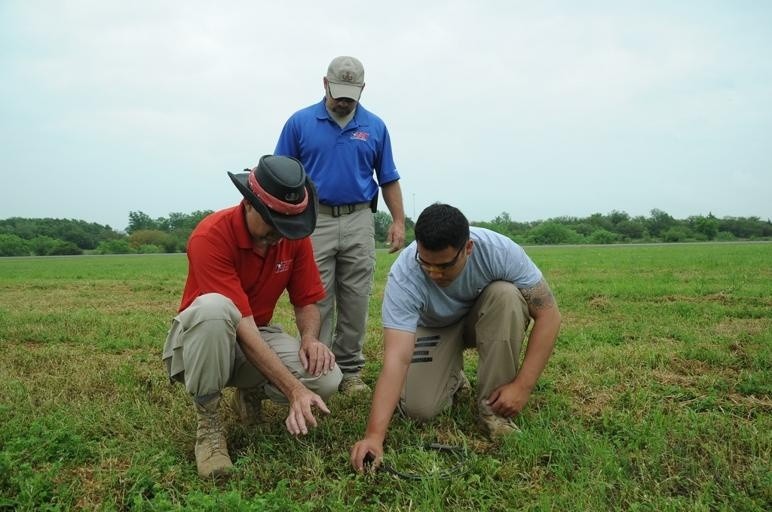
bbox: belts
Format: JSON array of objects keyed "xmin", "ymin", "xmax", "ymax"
[{"xmin": 319, "ymin": 201, "xmax": 371, "ymax": 217}]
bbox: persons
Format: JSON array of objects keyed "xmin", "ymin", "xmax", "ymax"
[
  {"xmin": 349, "ymin": 203, "xmax": 562, "ymax": 475},
  {"xmin": 273, "ymin": 58, "xmax": 405, "ymax": 399},
  {"xmin": 161, "ymin": 155, "xmax": 346, "ymax": 483}
]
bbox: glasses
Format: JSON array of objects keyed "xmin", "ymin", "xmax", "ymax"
[{"xmin": 414, "ymin": 240, "xmax": 467, "ymax": 271}]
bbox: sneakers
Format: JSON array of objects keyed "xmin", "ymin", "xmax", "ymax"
[
  {"xmin": 480, "ymin": 413, "xmax": 521, "ymax": 441},
  {"xmin": 455, "ymin": 369, "xmax": 473, "ymax": 402},
  {"xmin": 340, "ymin": 377, "xmax": 372, "ymax": 396}
]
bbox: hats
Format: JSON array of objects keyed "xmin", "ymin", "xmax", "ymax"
[
  {"xmin": 225, "ymin": 154, "xmax": 320, "ymax": 241},
  {"xmin": 326, "ymin": 54, "xmax": 366, "ymax": 102}
]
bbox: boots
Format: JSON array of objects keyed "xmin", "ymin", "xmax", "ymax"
[
  {"xmin": 234, "ymin": 385, "xmax": 274, "ymax": 431},
  {"xmin": 193, "ymin": 397, "xmax": 235, "ymax": 479}
]
